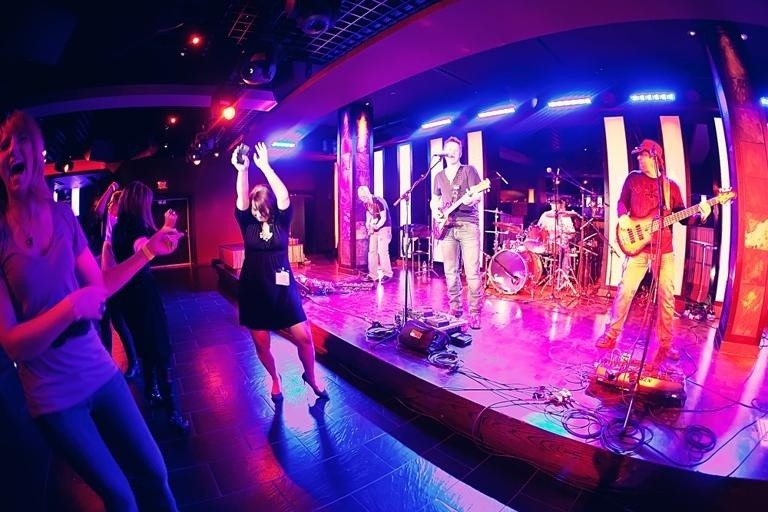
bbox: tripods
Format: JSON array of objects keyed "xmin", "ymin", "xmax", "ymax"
[
  {"xmin": 605, "ymin": 155, "xmax": 663, "ymax": 440},
  {"xmin": 480, "ymin": 177, "xmax": 596, "ymax": 313},
  {"xmin": 369, "ymin": 159, "xmax": 443, "ymax": 351}
]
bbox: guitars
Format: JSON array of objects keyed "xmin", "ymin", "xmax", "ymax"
[
  {"xmin": 616, "ymin": 187, "xmax": 735, "ymax": 256},
  {"xmin": 433, "ymin": 178, "xmax": 491, "ymax": 240}
]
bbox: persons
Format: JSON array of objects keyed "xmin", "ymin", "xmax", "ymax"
[
  {"xmin": 537, "ymin": 195, "xmax": 575, "ymax": 286},
  {"xmin": 229, "ymin": 140, "xmax": 330, "ymax": 406},
  {"xmin": 89, "ymin": 179, "xmax": 117, "ymax": 219},
  {"xmin": 595, "ymin": 138, "xmax": 712, "ymax": 363},
  {"xmin": 101, "ymin": 189, "xmax": 140, "ymax": 386},
  {"xmin": 1, "ymin": 110, "xmax": 184, "ymax": 511},
  {"xmin": 109, "ymin": 179, "xmax": 191, "ymax": 441},
  {"xmin": 427, "ymin": 134, "xmax": 485, "ymax": 330},
  {"xmin": 356, "ymin": 184, "xmax": 393, "ymax": 283}
]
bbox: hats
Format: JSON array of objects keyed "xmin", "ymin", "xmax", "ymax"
[{"xmin": 630, "ymin": 138, "xmax": 663, "ymax": 163}]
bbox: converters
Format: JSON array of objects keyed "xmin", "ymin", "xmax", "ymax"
[{"xmin": 552, "ymin": 387, "xmax": 573, "ymax": 402}]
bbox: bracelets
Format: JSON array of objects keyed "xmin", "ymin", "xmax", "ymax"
[{"xmin": 140, "ymin": 244, "xmax": 155, "ymax": 260}]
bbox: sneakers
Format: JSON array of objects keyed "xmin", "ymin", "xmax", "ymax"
[
  {"xmin": 447, "ymin": 309, "xmax": 482, "ymax": 329},
  {"xmin": 595, "ymin": 333, "xmax": 618, "ymax": 349},
  {"xmin": 656, "ymin": 345, "xmax": 683, "ymax": 361},
  {"xmin": 362, "ymin": 274, "xmax": 375, "ymax": 282},
  {"xmin": 380, "ymin": 275, "xmax": 393, "ymax": 283}
]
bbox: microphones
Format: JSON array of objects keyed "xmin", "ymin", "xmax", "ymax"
[
  {"xmin": 546, "ymin": 167, "xmax": 558, "ymax": 177},
  {"xmin": 649, "ymin": 143, "xmax": 657, "ymax": 157},
  {"xmin": 432, "ymin": 152, "xmax": 455, "ymax": 158}
]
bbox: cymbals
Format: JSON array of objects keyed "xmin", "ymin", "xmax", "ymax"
[
  {"xmin": 545, "ymin": 211, "xmax": 576, "ymax": 217},
  {"xmin": 493, "ymin": 221, "xmax": 521, "ymax": 234}
]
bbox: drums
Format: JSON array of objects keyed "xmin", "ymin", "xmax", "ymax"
[
  {"xmin": 488, "ymin": 249, "xmax": 542, "ymax": 296},
  {"xmin": 523, "ymin": 225, "xmax": 550, "ymax": 254}
]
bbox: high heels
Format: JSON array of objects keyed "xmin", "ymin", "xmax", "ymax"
[
  {"xmin": 302, "ymin": 371, "xmax": 329, "ymax": 398},
  {"xmin": 271, "ymin": 372, "xmax": 284, "ymax": 401},
  {"xmin": 125, "ymin": 362, "xmax": 190, "ymax": 438}
]
